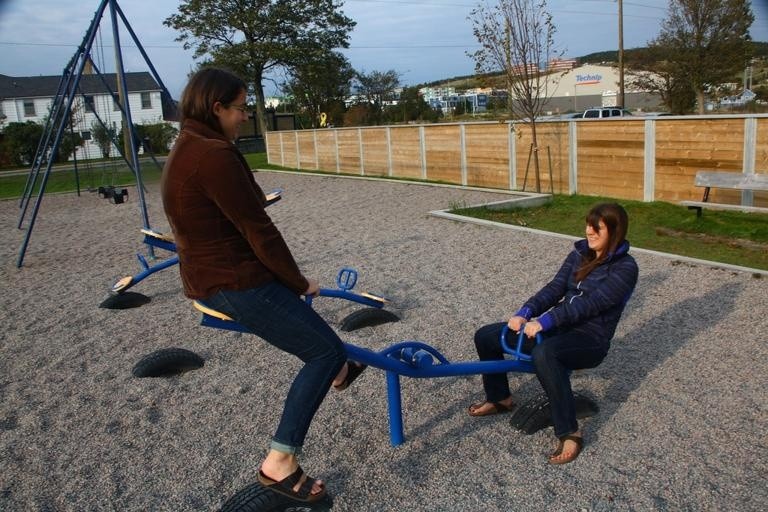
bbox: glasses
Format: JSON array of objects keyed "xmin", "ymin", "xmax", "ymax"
[{"xmin": 228, "ymin": 103, "xmax": 249, "ymax": 113}]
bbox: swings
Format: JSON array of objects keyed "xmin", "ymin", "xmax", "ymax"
[{"xmin": 67, "ymin": 24, "xmax": 129, "ymax": 204}]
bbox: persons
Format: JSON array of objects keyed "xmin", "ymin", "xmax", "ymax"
[
  {"xmin": 467, "ymin": 203, "xmax": 639, "ymax": 466},
  {"xmin": 157, "ymin": 69, "xmax": 368, "ymax": 503}
]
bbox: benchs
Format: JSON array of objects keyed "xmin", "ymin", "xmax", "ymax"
[{"xmin": 678, "ymin": 168, "xmax": 768, "ymax": 219}]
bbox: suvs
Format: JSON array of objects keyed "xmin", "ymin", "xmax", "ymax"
[{"xmin": 583, "ymin": 106, "xmax": 633, "ymax": 118}]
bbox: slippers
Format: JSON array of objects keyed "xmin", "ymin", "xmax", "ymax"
[
  {"xmin": 334, "ymin": 357, "xmax": 367, "ymax": 392},
  {"xmin": 258, "ymin": 466, "xmax": 327, "ymax": 502},
  {"xmin": 468, "ymin": 397, "xmax": 514, "ymax": 416},
  {"xmin": 550, "ymin": 433, "xmax": 584, "ymax": 465}
]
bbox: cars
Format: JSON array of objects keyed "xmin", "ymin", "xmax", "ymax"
[
  {"xmin": 638, "ymin": 112, "xmax": 676, "ymax": 117},
  {"xmin": 560, "ymin": 112, "xmax": 583, "ymax": 119}
]
